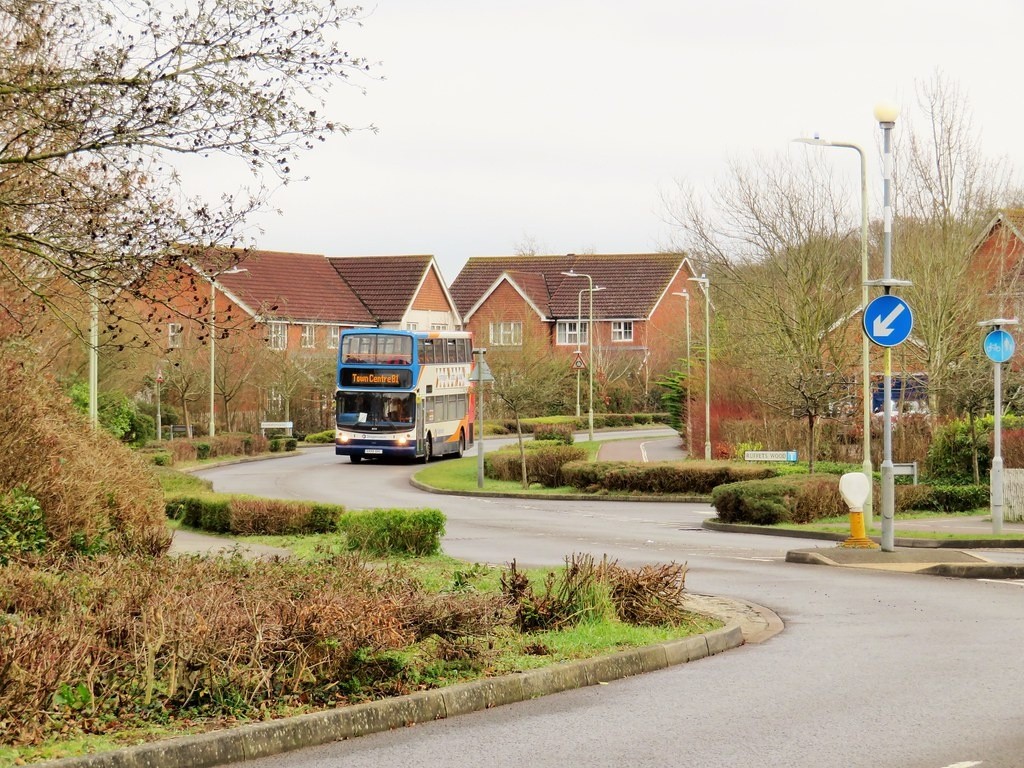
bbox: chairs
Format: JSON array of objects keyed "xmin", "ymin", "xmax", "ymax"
[{"xmin": 345, "ymin": 353, "xmax": 464, "ymax": 364}]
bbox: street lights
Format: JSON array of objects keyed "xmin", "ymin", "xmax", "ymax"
[
  {"xmin": 863, "ymin": 103, "xmax": 913, "ymax": 551},
  {"xmin": 687, "ymin": 276, "xmax": 711, "ymax": 463},
  {"xmin": 793, "ymin": 134, "xmax": 873, "ymax": 532},
  {"xmin": 559, "ymin": 271, "xmax": 593, "ymax": 441},
  {"xmin": 671, "ymin": 291, "xmax": 692, "ymax": 459},
  {"xmin": 574, "ymin": 286, "xmax": 606, "ymax": 418},
  {"xmin": 208, "ymin": 268, "xmax": 248, "ymax": 437}
]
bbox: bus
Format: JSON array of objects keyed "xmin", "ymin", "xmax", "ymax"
[{"xmin": 332, "ymin": 329, "xmax": 475, "ymax": 464}]
